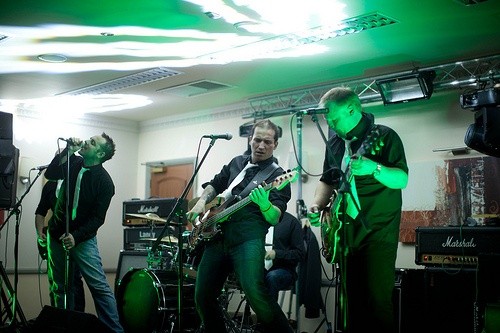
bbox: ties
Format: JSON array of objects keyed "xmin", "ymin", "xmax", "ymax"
[
  {"xmin": 71, "ymin": 166, "xmax": 90, "ymax": 220},
  {"xmin": 56, "ymin": 180, "xmax": 64, "ymax": 198},
  {"xmin": 342, "ymin": 136, "xmax": 361, "ymax": 219},
  {"xmin": 264, "ymin": 226, "xmax": 274, "ymax": 271},
  {"xmin": 225, "ymin": 161, "xmax": 259, "ymax": 201}
]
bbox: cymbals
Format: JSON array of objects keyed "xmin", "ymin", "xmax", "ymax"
[
  {"xmin": 140, "ymin": 235, "xmax": 182, "ymax": 242},
  {"xmin": 187, "ymin": 196, "xmax": 228, "ymax": 214},
  {"xmin": 127, "ymin": 212, "xmax": 178, "ymax": 224}
]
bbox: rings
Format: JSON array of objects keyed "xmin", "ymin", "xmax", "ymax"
[{"xmin": 70, "ymin": 243, "xmax": 72, "ymax": 245}]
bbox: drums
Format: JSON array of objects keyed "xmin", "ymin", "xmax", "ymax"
[
  {"xmin": 173, "ymin": 230, "xmax": 207, "ymax": 279},
  {"xmin": 224, "ymin": 270, "xmax": 242, "ymax": 293},
  {"xmin": 116, "ymin": 268, "xmax": 201, "ymax": 333}
]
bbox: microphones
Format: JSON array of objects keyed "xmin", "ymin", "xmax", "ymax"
[
  {"xmin": 297, "ymin": 107, "xmax": 330, "ymax": 114},
  {"xmin": 202, "ymin": 132, "xmax": 233, "ymax": 140},
  {"xmin": 31, "ymin": 164, "xmax": 49, "ymax": 170},
  {"xmin": 58, "ymin": 138, "xmax": 85, "ymax": 148}
]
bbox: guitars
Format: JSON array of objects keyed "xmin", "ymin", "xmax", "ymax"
[
  {"xmin": 187, "ymin": 168, "xmax": 301, "ymax": 252},
  {"xmin": 37, "ymin": 227, "xmax": 46, "ymax": 260},
  {"xmin": 321, "ymin": 129, "xmax": 385, "ymax": 266}
]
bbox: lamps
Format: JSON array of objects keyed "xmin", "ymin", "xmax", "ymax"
[
  {"xmin": 379, "ymin": 70, "xmax": 436, "ymax": 106},
  {"xmin": 459, "ymin": 71, "xmax": 500, "ymax": 158}
]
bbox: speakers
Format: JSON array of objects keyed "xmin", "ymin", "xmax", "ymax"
[
  {"xmin": 114, "ymin": 250, "xmax": 169, "ymax": 286},
  {"xmin": 392, "ymin": 267, "xmax": 480, "ymax": 332},
  {"xmin": 34, "ymin": 304, "xmax": 118, "ymax": 333}
]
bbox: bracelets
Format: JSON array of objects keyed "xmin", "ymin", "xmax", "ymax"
[{"xmin": 260, "ymin": 203, "xmax": 272, "ymax": 212}]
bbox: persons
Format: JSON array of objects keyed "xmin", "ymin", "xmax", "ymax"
[
  {"xmin": 34, "ymin": 131, "xmax": 125, "ymax": 333},
  {"xmin": 179, "ymin": 119, "xmax": 304, "ymax": 333},
  {"xmin": 307, "ymin": 87, "xmax": 409, "ymax": 333}
]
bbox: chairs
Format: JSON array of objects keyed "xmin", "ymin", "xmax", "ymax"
[{"xmin": 282, "ymin": 224, "xmax": 332, "ymax": 333}]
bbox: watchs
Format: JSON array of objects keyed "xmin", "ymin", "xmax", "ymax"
[{"xmin": 373, "ymin": 163, "xmax": 381, "ymax": 177}]
